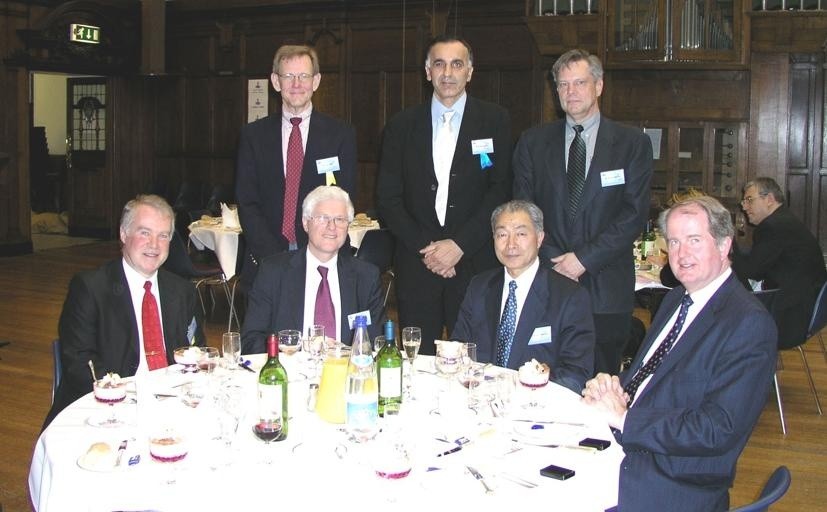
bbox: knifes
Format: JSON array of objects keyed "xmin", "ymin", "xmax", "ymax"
[{"xmin": 467, "ymin": 465, "xmax": 493, "ymax": 493}]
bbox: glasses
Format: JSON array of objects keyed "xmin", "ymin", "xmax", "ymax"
[{"xmin": 277, "ymin": 72, "xmax": 313, "ymax": 81}]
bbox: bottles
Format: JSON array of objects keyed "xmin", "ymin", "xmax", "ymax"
[
  {"xmin": 641, "ymin": 219, "xmax": 657, "ymax": 260},
  {"xmin": 256, "ymin": 332, "xmax": 290, "ymax": 444},
  {"xmin": 376, "ymin": 317, "xmax": 406, "ymax": 418},
  {"xmin": 309, "ymin": 383, "xmax": 319, "ymax": 410},
  {"xmin": 343, "ymin": 314, "xmax": 378, "ymax": 442}
]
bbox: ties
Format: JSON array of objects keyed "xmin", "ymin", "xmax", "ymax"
[
  {"xmin": 314, "ymin": 266, "xmax": 336, "ymax": 340},
  {"xmin": 623, "ymin": 295, "xmax": 693, "ymax": 407},
  {"xmin": 496, "ymin": 281, "xmax": 517, "ymax": 367},
  {"xmin": 567, "ymin": 125, "xmax": 586, "ymax": 225},
  {"xmin": 142, "ymin": 281, "xmax": 169, "ymax": 371},
  {"xmin": 282, "ymin": 118, "xmax": 304, "ymax": 242},
  {"xmin": 434, "ymin": 111, "xmax": 454, "ymax": 227}
]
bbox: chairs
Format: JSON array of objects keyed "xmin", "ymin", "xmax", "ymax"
[
  {"xmin": 163, "ymin": 229, "xmax": 240, "ymax": 330},
  {"xmin": 729, "ymin": 465, "xmax": 791, "ymax": 512},
  {"xmin": 52, "ymin": 339, "xmax": 64, "ymax": 406},
  {"xmin": 779, "ymin": 281, "xmax": 827, "ymax": 415},
  {"xmin": 750, "ymin": 288, "xmax": 786, "ymax": 434},
  {"xmin": 357, "ymin": 229, "xmax": 395, "ymax": 308}
]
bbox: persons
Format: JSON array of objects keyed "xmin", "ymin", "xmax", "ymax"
[
  {"xmin": 239, "ymin": 184, "xmax": 392, "ymax": 355},
  {"xmin": 730, "ymin": 179, "xmax": 825, "ymax": 346},
  {"xmin": 376, "ymin": 37, "xmax": 510, "ymax": 354},
  {"xmin": 508, "ymin": 47, "xmax": 650, "ymax": 377},
  {"xmin": 577, "ymin": 191, "xmax": 777, "ymax": 511},
  {"xmin": 40, "ymin": 193, "xmax": 210, "ymax": 436},
  {"xmin": 235, "ymin": 44, "xmax": 358, "ymax": 333},
  {"xmin": 449, "ymin": 202, "xmax": 594, "ymax": 398}
]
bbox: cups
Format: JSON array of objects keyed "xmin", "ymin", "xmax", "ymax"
[
  {"xmin": 92, "ymin": 384, "xmax": 127, "ymax": 405},
  {"xmin": 249, "ymin": 408, "xmax": 285, "ymax": 463},
  {"xmin": 148, "ymin": 440, "xmax": 188, "ymax": 464},
  {"xmin": 373, "ymin": 335, "xmax": 398, "ymax": 353},
  {"xmin": 278, "ymin": 329, "xmax": 302, "ymax": 358},
  {"xmin": 162, "ymin": 331, "xmax": 241, "ymax": 431},
  {"xmin": 307, "ymin": 324, "xmax": 326, "ymax": 356},
  {"xmin": 373, "ymin": 458, "xmax": 413, "ymax": 480},
  {"xmin": 519, "ymin": 369, "xmax": 551, "ymax": 388}
]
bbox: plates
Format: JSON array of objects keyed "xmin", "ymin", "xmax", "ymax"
[
  {"xmin": 86, "ymin": 415, "xmax": 124, "ymax": 428},
  {"xmin": 77, "ymin": 454, "xmax": 118, "ymax": 471}
]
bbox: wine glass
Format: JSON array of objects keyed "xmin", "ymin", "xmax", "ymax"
[
  {"xmin": 419, "ymin": 340, "xmax": 516, "ymax": 450},
  {"xmin": 401, "ymin": 326, "xmax": 423, "ymax": 375}
]
